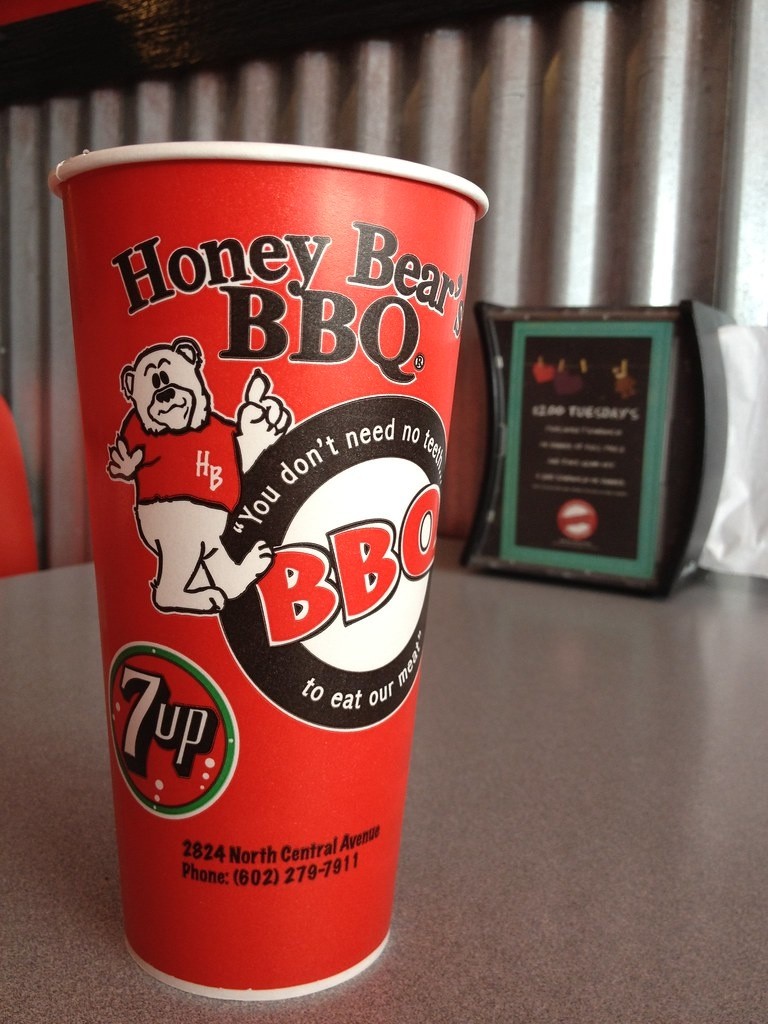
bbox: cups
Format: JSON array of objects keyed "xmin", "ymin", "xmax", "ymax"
[{"xmin": 44, "ymin": 140, "xmax": 489, "ymax": 1000}]
